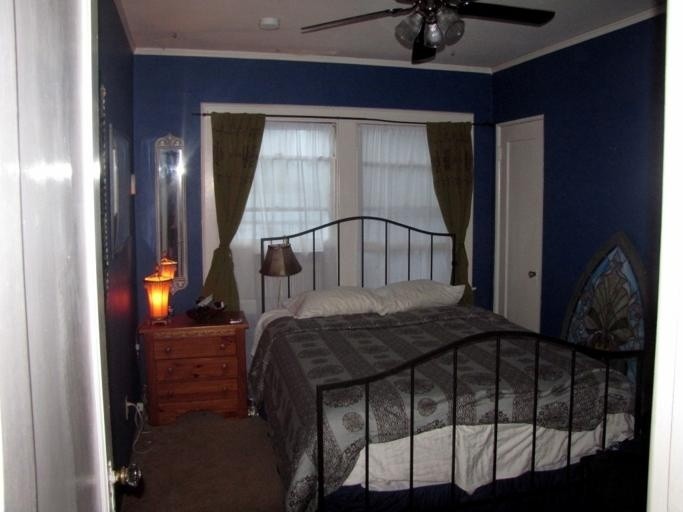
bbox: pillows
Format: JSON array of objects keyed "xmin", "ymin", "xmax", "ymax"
[{"xmin": 281, "ymin": 278, "xmax": 468, "ymax": 319}]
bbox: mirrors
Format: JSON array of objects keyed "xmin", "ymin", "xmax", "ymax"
[{"xmin": 155, "ymin": 131, "xmax": 189, "ymax": 299}]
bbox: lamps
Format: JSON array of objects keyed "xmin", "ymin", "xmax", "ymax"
[
  {"xmin": 394, "ymin": 5, "xmax": 465, "ymax": 50},
  {"xmin": 143, "ymin": 264, "xmax": 172, "ymax": 326},
  {"xmin": 260, "ymin": 243, "xmax": 303, "ymax": 309},
  {"xmin": 160, "ymin": 256, "xmax": 178, "ymax": 278}
]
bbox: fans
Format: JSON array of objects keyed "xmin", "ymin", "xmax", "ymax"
[{"xmin": 299, "ymin": 1, "xmax": 556, "ymax": 65}]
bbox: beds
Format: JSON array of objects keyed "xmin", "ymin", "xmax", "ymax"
[{"xmin": 260, "ymin": 215, "xmax": 648, "ymax": 512}]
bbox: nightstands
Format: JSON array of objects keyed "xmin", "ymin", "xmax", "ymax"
[{"xmin": 136, "ymin": 311, "xmax": 251, "ymax": 427}]
